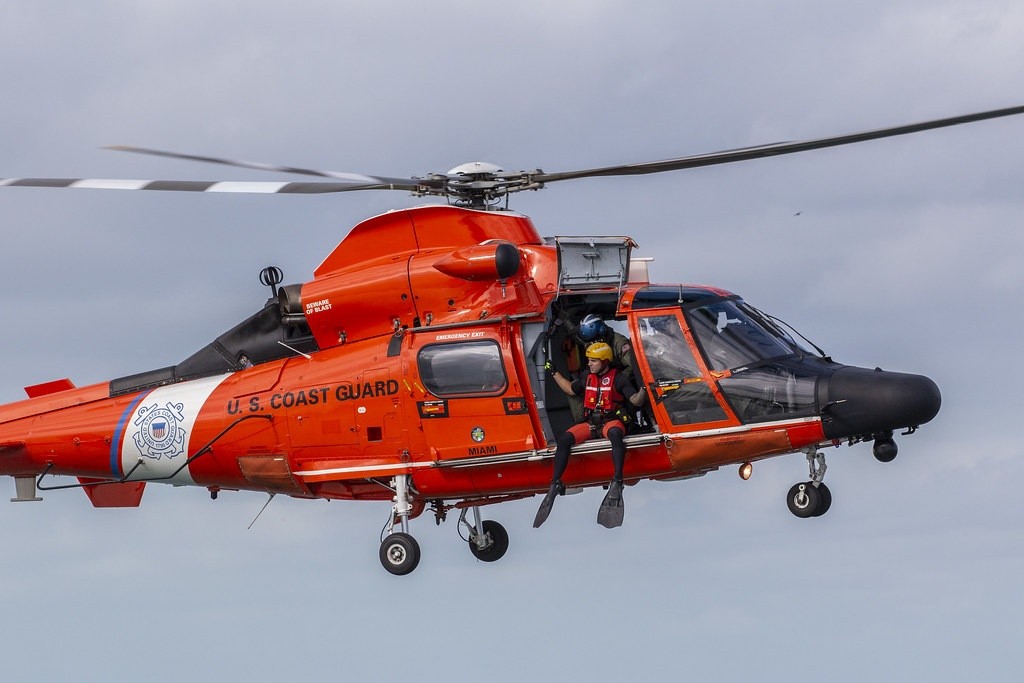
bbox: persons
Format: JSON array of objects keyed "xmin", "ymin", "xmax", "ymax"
[
  {"xmin": 533, "ymin": 342, "xmax": 648, "ymax": 529},
  {"xmin": 646, "ymin": 303, "xmax": 723, "ymax": 404},
  {"xmin": 553, "ymin": 313, "xmax": 638, "ymax": 392}
]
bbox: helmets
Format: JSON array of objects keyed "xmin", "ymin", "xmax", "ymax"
[
  {"xmin": 579, "ymin": 314, "xmax": 607, "ymax": 342},
  {"xmin": 585, "ymin": 342, "xmax": 613, "ymax": 362}
]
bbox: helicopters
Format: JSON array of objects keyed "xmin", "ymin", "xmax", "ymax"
[{"xmin": 0, "ymin": 101, "xmax": 1024, "ymax": 577}]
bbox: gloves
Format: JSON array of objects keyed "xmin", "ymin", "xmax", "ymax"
[{"xmin": 544, "ymin": 359, "xmax": 557, "ymax": 376}]
